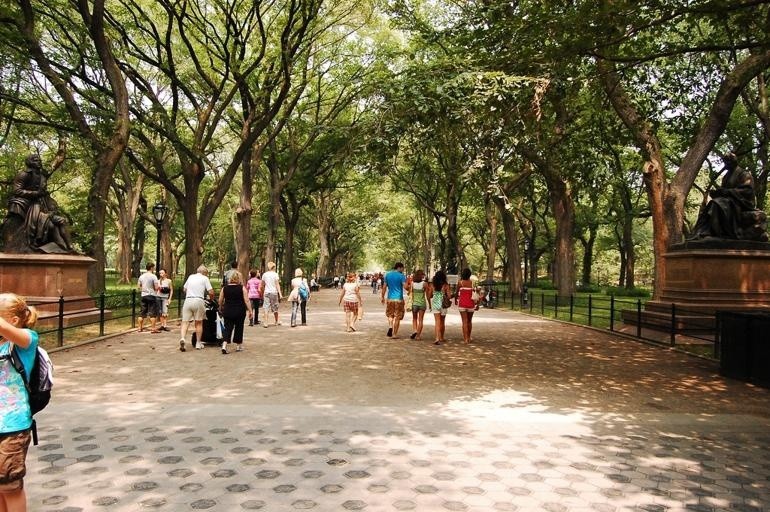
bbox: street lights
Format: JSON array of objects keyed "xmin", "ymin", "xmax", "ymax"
[
  {"xmin": 522, "ymin": 240, "xmax": 530, "ymax": 288},
  {"xmin": 426, "ymin": 261, "xmax": 430, "ymax": 281},
  {"xmin": 459, "ymin": 252, "xmax": 464, "ymax": 277},
  {"xmin": 275, "ymin": 246, "xmax": 282, "ymax": 272},
  {"xmin": 152, "ymin": 201, "xmax": 168, "ymax": 279},
  {"xmin": 315, "ymin": 257, "xmax": 318, "ymax": 281}
]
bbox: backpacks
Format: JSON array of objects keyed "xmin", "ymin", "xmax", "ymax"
[{"xmin": 7, "ymin": 336, "xmax": 54, "ymax": 447}]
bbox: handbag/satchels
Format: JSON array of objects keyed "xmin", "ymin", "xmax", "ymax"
[
  {"xmin": 441, "ymin": 284, "xmax": 452, "ymax": 310},
  {"xmin": 215, "ymin": 311, "xmax": 226, "ymax": 339},
  {"xmin": 299, "ymin": 277, "xmax": 310, "ymax": 299},
  {"xmin": 358, "ymin": 303, "xmax": 364, "ymax": 321},
  {"xmin": 405, "ymin": 281, "xmax": 414, "ymax": 313},
  {"xmin": 159, "ymin": 286, "xmax": 171, "ymax": 294},
  {"xmin": 470, "ymin": 280, "xmax": 481, "ymax": 303}
]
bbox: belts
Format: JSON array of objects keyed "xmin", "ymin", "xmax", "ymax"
[{"xmin": 187, "ymin": 296, "xmax": 203, "ymax": 299}]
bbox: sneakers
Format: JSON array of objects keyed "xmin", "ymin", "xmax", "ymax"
[
  {"xmin": 158, "ymin": 326, "xmax": 164, "ymax": 331},
  {"xmin": 164, "ymin": 327, "xmax": 170, "ymax": 331},
  {"xmin": 178, "ymin": 339, "xmax": 245, "ymax": 353}
]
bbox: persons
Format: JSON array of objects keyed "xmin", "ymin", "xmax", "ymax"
[
  {"xmin": 0, "ymin": 291, "xmax": 40, "ymax": 512},
  {"xmin": 8, "ymin": 154, "xmax": 74, "ymax": 250},
  {"xmin": 691, "ymin": 152, "xmax": 755, "ymax": 237}
]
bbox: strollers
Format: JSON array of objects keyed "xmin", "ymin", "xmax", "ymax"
[{"xmin": 191, "ymin": 297, "xmax": 227, "ymax": 347}]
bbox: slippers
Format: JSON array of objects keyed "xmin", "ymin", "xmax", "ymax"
[{"xmin": 348, "ymin": 326, "xmax": 473, "ymax": 344}]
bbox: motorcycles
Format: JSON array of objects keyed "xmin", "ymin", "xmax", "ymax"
[{"xmin": 480, "ymin": 289, "xmax": 498, "ymax": 308}]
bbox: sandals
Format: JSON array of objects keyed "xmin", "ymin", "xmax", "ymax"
[
  {"xmin": 138, "ymin": 328, "xmax": 146, "ymax": 332},
  {"xmin": 151, "ymin": 328, "xmax": 161, "ymax": 333},
  {"xmin": 248, "ymin": 321, "xmax": 307, "ymax": 328}
]
